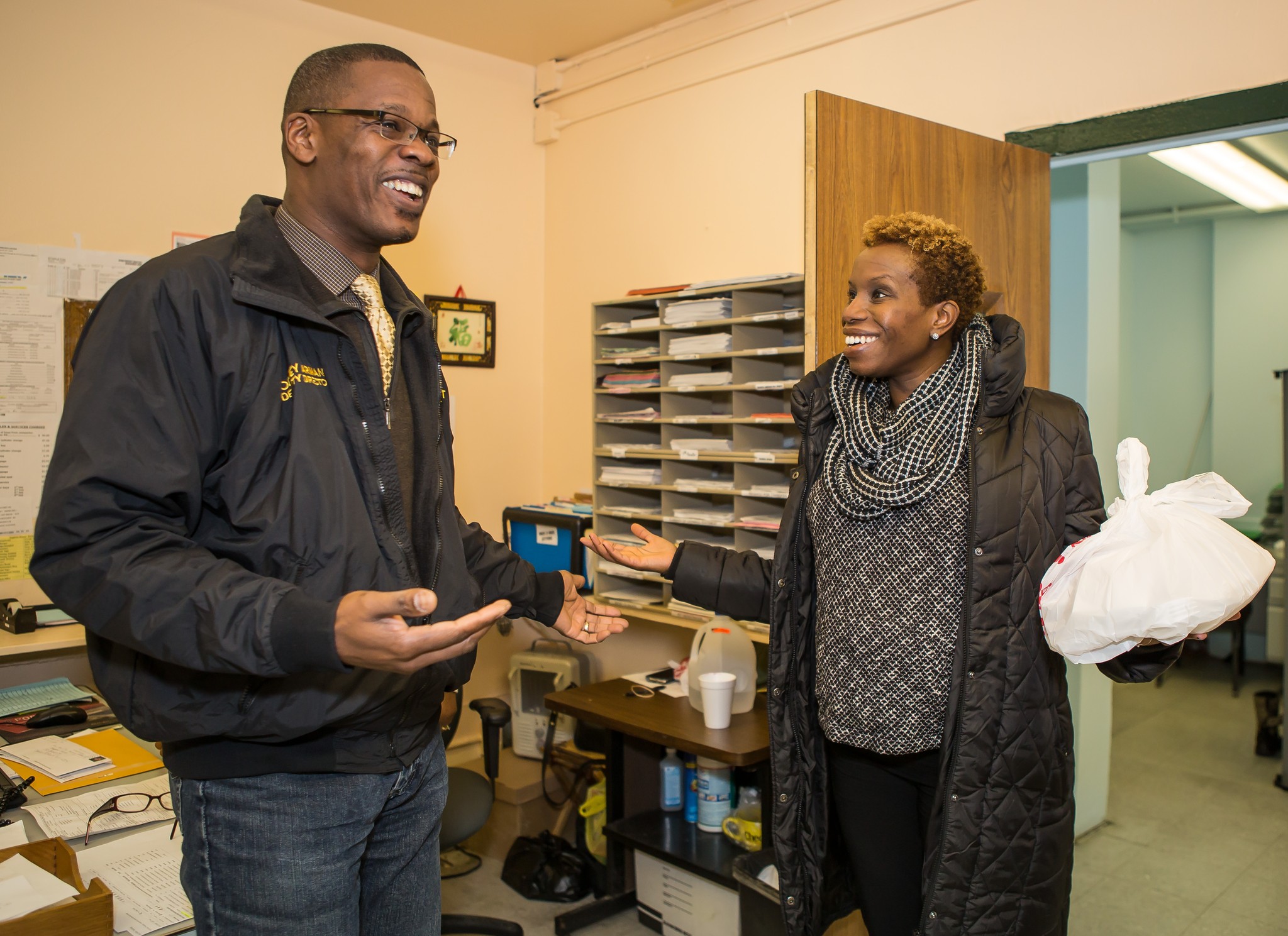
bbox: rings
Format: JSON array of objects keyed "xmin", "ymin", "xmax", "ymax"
[
  {"xmin": 584, "ymin": 621, "xmax": 589, "ymax": 632},
  {"xmin": 1142, "ymin": 637, "xmax": 1152, "ymax": 646}
]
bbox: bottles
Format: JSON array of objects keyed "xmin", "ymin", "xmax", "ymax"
[
  {"xmin": 688, "ymin": 610, "xmax": 756, "ymax": 714},
  {"xmin": 699, "ymin": 752, "xmax": 732, "ymax": 833},
  {"xmin": 683, "ymin": 752, "xmax": 702, "ymax": 823},
  {"xmin": 659, "ymin": 748, "xmax": 685, "ymax": 812}
]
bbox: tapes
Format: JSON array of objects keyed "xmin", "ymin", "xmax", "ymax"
[{"xmin": 2, "ymin": 601, "xmax": 23, "ymax": 628}]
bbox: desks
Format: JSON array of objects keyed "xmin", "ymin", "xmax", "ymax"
[
  {"xmin": 542, "ymin": 668, "xmax": 784, "ymax": 935},
  {"xmin": 0, "ymin": 617, "xmax": 121, "ymax": 746},
  {"xmin": 0, "ymin": 727, "xmax": 197, "ymax": 936}
]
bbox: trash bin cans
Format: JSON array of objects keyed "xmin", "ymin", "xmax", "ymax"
[{"xmin": 1254, "ymin": 689, "xmax": 1283, "ymax": 757}]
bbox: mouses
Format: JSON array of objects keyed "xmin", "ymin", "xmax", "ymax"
[{"xmin": 25, "ymin": 704, "xmax": 87, "ymax": 728}]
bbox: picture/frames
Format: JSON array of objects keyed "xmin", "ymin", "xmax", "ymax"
[{"xmin": 423, "ymin": 295, "xmax": 497, "ymax": 367}]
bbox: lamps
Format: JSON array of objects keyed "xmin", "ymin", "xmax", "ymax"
[{"xmin": 1146, "ymin": 140, "xmax": 1287, "ymax": 214}]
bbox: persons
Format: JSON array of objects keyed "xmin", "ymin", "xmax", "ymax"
[
  {"xmin": 578, "ymin": 211, "xmax": 1244, "ymax": 936},
  {"xmin": 29, "ymin": 43, "xmax": 629, "ymax": 936}
]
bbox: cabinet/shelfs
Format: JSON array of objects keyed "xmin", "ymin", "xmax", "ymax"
[
  {"xmin": 632, "ymin": 848, "xmax": 755, "ymax": 936},
  {"xmin": 590, "ymin": 274, "xmax": 806, "ymax": 685}
]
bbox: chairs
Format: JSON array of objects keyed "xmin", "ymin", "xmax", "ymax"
[{"xmin": 439, "ymin": 685, "xmax": 493, "ymax": 881}]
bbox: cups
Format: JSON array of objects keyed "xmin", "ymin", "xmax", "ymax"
[
  {"xmin": 722, "ymin": 802, "xmax": 762, "ymax": 851},
  {"xmin": 698, "ymin": 672, "xmax": 737, "ymax": 729}
]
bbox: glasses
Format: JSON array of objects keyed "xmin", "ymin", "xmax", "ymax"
[{"xmin": 294, "ymin": 107, "xmax": 458, "ymax": 161}]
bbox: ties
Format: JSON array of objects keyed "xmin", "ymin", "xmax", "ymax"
[{"xmin": 351, "ymin": 274, "xmax": 395, "ymax": 404}]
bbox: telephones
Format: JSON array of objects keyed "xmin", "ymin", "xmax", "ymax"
[{"xmin": 0, "ymin": 767, "xmax": 28, "ymax": 812}]
